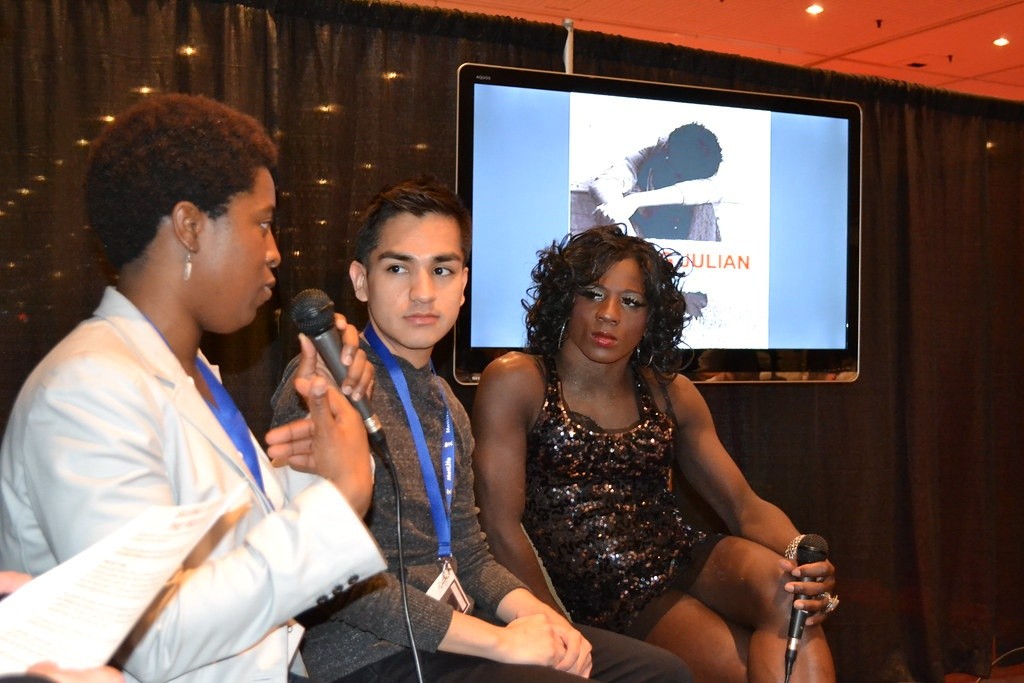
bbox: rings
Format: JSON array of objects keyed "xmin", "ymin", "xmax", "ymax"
[{"xmin": 823, "ymin": 591, "xmax": 840, "ymax": 612}]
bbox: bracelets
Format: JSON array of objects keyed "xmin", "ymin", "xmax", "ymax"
[{"xmin": 784, "ymin": 534, "xmax": 805, "ymax": 559}]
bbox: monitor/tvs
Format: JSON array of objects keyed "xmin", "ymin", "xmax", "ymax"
[{"xmin": 450, "ymin": 63, "xmax": 864, "ymax": 388}]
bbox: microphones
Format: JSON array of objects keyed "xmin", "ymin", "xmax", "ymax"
[
  {"xmin": 784, "ymin": 534, "xmax": 828, "ymax": 675},
  {"xmin": 287, "ymin": 288, "xmax": 393, "ymax": 463}
]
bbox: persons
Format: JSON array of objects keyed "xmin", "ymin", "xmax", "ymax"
[
  {"xmin": 468, "ymin": 223, "xmax": 841, "ymax": 683},
  {"xmin": 589, "ymin": 121, "xmax": 725, "ymax": 242},
  {"xmin": 0, "ymin": 89, "xmax": 390, "ymax": 683},
  {"xmin": 269, "ymin": 180, "xmax": 692, "ymax": 683}
]
